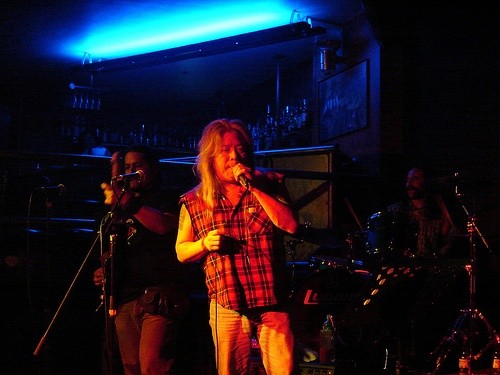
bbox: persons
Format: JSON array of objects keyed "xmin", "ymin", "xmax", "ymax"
[
  {"xmin": 93, "ymin": 141, "xmax": 180, "ymax": 374},
  {"xmin": 383, "ymin": 166, "xmax": 459, "ymax": 256},
  {"xmin": 175, "ymin": 119, "xmax": 300, "ymax": 375}
]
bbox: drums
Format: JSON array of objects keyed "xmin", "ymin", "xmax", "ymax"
[
  {"xmin": 288, "ymin": 256, "xmax": 461, "ymax": 362},
  {"xmin": 367, "ymin": 212, "xmax": 419, "ymax": 248}
]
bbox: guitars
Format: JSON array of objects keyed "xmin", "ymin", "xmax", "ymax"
[{"xmin": 96, "ymin": 151, "xmax": 123, "ymax": 314}]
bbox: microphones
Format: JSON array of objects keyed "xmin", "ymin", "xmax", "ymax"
[
  {"xmin": 238, "ymin": 175, "xmax": 248, "ymax": 188},
  {"xmin": 111, "ymin": 170, "xmax": 145, "ymax": 182},
  {"xmin": 35, "ymin": 183, "xmax": 65, "ymax": 193}
]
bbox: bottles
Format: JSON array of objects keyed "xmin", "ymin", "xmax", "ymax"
[
  {"xmin": 57, "ymin": 117, "xmax": 202, "ymax": 151},
  {"xmin": 458, "ymin": 352, "xmax": 469, "ymax": 375},
  {"xmin": 72, "ymin": 94, "xmax": 101, "ymax": 109},
  {"xmin": 247, "ymin": 98, "xmax": 309, "ymax": 151},
  {"xmin": 493, "ymin": 351, "xmax": 500, "ymax": 375}
]
